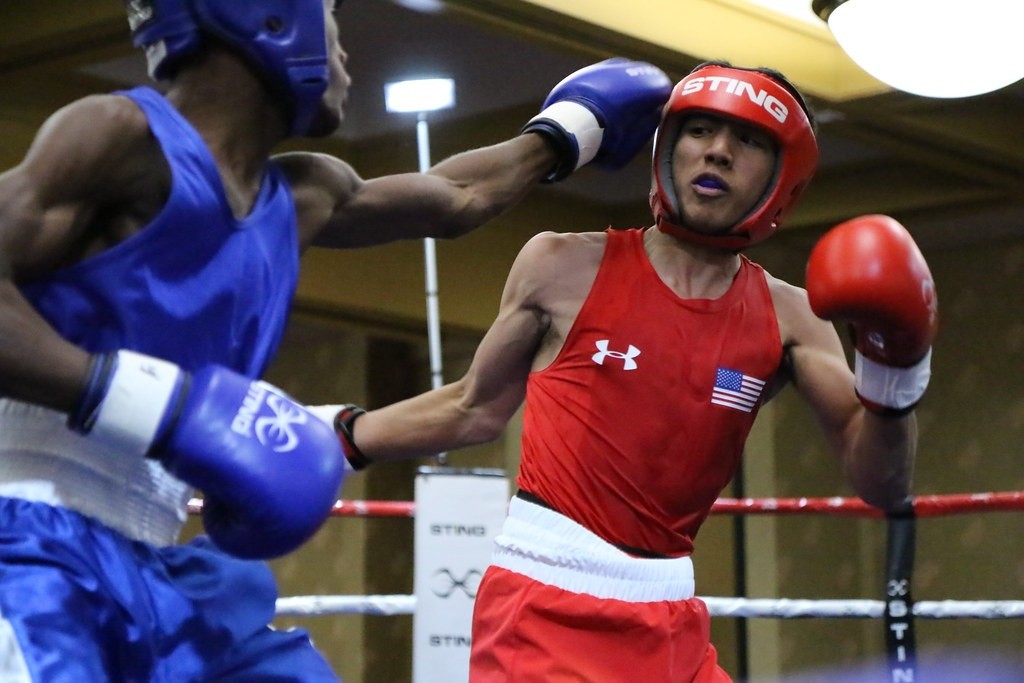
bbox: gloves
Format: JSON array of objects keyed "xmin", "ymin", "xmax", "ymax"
[
  {"xmin": 65, "ymin": 346, "xmax": 346, "ymax": 562},
  {"xmin": 524, "ymin": 56, "xmax": 674, "ymax": 185},
  {"xmin": 805, "ymin": 214, "xmax": 938, "ymax": 419}
]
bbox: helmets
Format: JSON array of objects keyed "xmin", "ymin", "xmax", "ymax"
[
  {"xmin": 128, "ymin": 0, "xmax": 332, "ymax": 134},
  {"xmin": 647, "ymin": 62, "xmax": 822, "ymax": 253}
]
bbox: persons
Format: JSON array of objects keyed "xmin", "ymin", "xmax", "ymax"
[
  {"xmin": 0, "ymin": 0, "xmax": 674, "ymax": 683},
  {"xmin": 305, "ymin": 59, "xmax": 939, "ymax": 683}
]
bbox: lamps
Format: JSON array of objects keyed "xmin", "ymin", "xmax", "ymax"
[
  {"xmin": 384, "ymin": 78, "xmax": 456, "ymax": 113},
  {"xmin": 812, "ymin": 0, "xmax": 1024, "ymax": 99}
]
